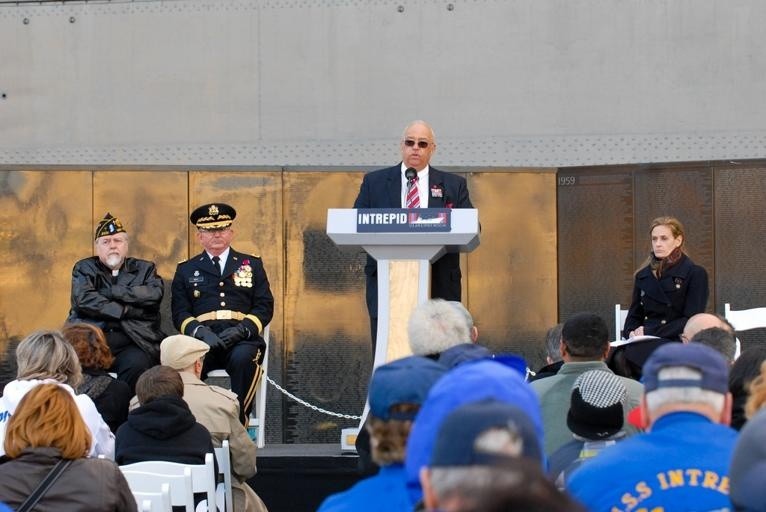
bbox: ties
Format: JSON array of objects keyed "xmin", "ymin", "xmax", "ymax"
[
  {"xmin": 406, "ymin": 176, "xmax": 421, "ymax": 208},
  {"xmin": 212, "ymin": 256, "xmax": 221, "ymax": 277}
]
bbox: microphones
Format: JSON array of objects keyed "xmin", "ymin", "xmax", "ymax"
[{"xmin": 404, "ymin": 164, "xmax": 419, "ymax": 185}]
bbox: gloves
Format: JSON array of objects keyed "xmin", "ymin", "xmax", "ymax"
[
  {"xmin": 195, "ymin": 326, "xmax": 228, "ymax": 351},
  {"xmin": 218, "ymin": 323, "xmax": 247, "ymax": 348}
]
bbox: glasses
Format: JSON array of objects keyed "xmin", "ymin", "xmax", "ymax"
[
  {"xmin": 405, "ymin": 141, "xmax": 428, "ymax": 148},
  {"xmin": 199, "ymin": 227, "xmax": 230, "ymax": 232}
]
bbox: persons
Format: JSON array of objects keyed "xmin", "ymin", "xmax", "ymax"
[
  {"xmin": 609, "ymin": 216, "xmax": 708, "ymax": 380},
  {"xmin": 0, "ymin": 323, "xmax": 268, "ymax": 512},
  {"xmin": 354, "ymin": 119, "xmax": 482, "ymax": 370},
  {"xmin": 63, "ymin": 215, "xmax": 166, "ymax": 412},
  {"xmin": 172, "ymin": 204, "xmax": 274, "ymax": 438},
  {"xmin": 319, "ymin": 301, "xmax": 764, "ymax": 512}
]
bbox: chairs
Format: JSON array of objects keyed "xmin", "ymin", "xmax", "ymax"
[
  {"xmin": 213, "ymin": 441, "xmax": 237, "ymax": 512},
  {"xmin": 208, "ymin": 325, "xmax": 270, "ymax": 448},
  {"xmin": 129, "ymin": 483, "xmax": 174, "ymax": 512},
  {"xmin": 121, "ymin": 467, "xmax": 197, "ymax": 512},
  {"xmin": 614, "ymin": 303, "xmax": 629, "ymax": 341},
  {"xmin": 142, "ymin": 500, "xmax": 152, "ymax": 512},
  {"xmin": 97, "ymin": 454, "xmax": 219, "ymax": 512},
  {"xmin": 725, "ymin": 303, "xmax": 766, "ymax": 331}
]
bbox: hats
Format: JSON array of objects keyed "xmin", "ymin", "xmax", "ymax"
[
  {"xmin": 159, "ymin": 334, "xmax": 211, "ymax": 369},
  {"xmin": 368, "ymin": 355, "xmax": 450, "ymax": 421},
  {"xmin": 95, "ymin": 212, "xmax": 126, "ymax": 240},
  {"xmin": 429, "ymin": 396, "xmax": 542, "ymax": 466},
  {"xmin": 436, "ymin": 341, "xmax": 492, "ymax": 369},
  {"xmin": 640, "ymin": 341, "xmax": 729, "ymax": 394},
  {"xmin": 190, "ymin": 203, "xmax": 236, "ymax": 229},
  {"xmin": 566, "ymin": 370, "xmax": 627, "ymax": 440}
]
